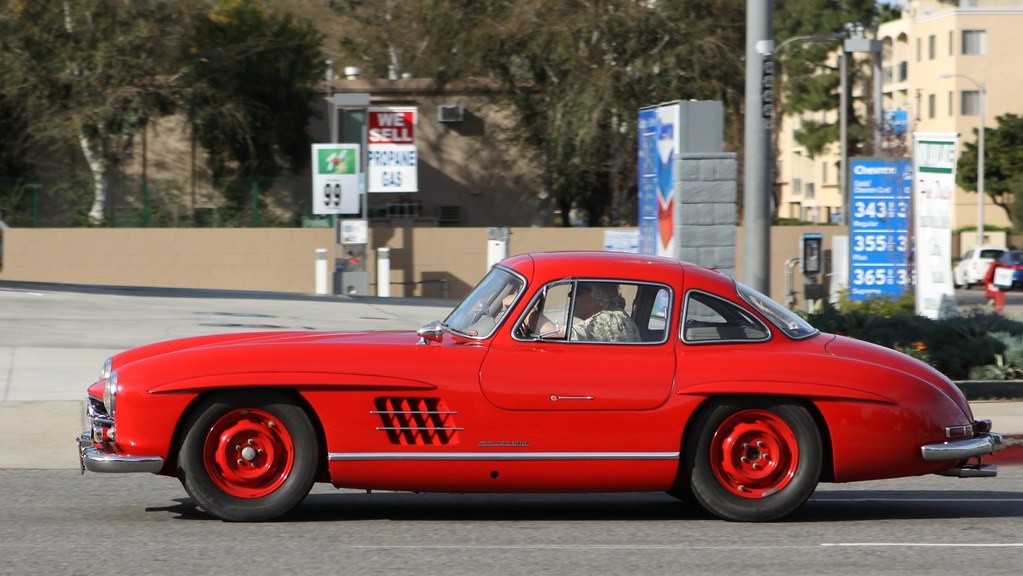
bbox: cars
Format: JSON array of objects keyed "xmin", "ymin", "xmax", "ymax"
[
  {"xmin": 953, "ymin": 246, "xmax": 1008, "ymax": 290},
  {"xmin": 999, "ymin": 250, "xmax": 1023, "ymax": 292}
]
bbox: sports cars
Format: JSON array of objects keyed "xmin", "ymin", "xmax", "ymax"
[{"xmin": 75, "ymin": 250, "xmax": 1005, "ymax": 523}]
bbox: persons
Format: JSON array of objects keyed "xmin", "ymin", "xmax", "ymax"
[{"xmin": 502, "ymin": 283, "xmax": 643, "ymax": 342}]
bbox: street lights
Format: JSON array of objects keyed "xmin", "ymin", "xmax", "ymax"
[{"xmin": 937, "ymin": 71, "xmax": 985, "ymax": 247}]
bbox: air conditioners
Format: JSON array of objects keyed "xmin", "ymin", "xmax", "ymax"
[{"xmin": 437, "ymin": 105, "xmax": 465, "ymax": 122}]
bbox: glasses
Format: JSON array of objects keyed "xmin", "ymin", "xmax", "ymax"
[{"xmin": 570, "ymin": 286, "xmax": 592, "ymax": 295}]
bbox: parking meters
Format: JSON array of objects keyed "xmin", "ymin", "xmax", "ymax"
[{"xmin": 799, "ymin": 233, "xmax": 822, "ymax": 314}]
